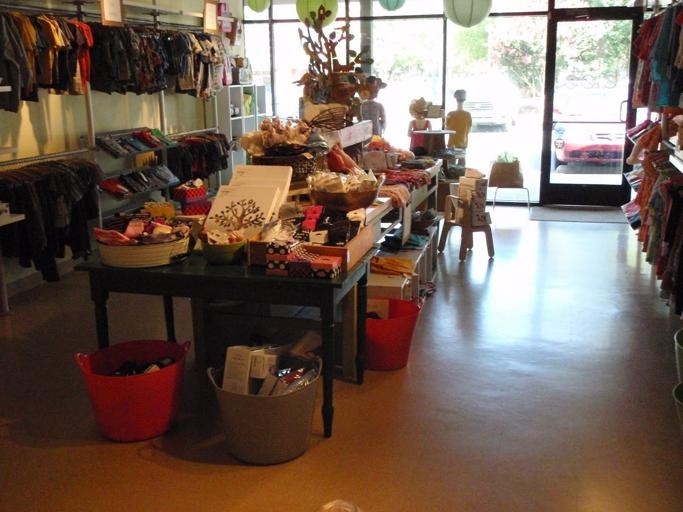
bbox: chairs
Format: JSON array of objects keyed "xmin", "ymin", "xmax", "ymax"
[{"xmin": 437, "ymin": 194, "xmax": 495, "ymax": 261}]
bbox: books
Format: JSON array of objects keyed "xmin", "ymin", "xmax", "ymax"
[
  {"xmin": 229, "ymin": 165, "xmax": 293, "ymax": 223},
  {"xmin": 194, "ymin": 185, "xmax": 279, "ymax": 252}
]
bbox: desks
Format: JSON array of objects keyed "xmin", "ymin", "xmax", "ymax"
[{"xmin": 74, "ymin": 153, "xmax": 443, "ymax": 439}]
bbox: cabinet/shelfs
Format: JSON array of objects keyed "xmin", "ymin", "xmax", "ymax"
[{"xmin": 205, "ymin": 84, "xmax": 267, "ymax": 186}]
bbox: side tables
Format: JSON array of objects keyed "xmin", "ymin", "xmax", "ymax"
[{"xmin": 412, "ymin": 129, "xmax": 457, "ymax": 179}]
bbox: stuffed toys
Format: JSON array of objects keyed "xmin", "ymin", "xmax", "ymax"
[{"xmin": 365, "ymin": 136, "xmax": 415, "ymax": 162}]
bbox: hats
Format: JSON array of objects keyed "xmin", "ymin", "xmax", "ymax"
[{"xmin": 409, "ymin": 97, "xmax": 432, "ymax": 120}]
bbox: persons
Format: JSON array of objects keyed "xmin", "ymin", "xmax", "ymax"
[
  {"xmin": 444, "ymin": 89, "xmax": 472, "ymax": 164},
  {"xmin": 407, "ymin": 96, "xmax": 431, "ymax": 154},
  {"xmin": 361, "ymin": 76, "xmax": 386, "ymax": 135}
]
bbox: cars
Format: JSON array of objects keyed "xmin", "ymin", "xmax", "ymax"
[{"xmin": 551, "ymin": 107, "xmax": 623, "ymax": 173}]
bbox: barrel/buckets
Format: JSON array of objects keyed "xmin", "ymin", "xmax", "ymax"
[
  {"xmin": 206, "ymin": 351, "xmax": 323, "ymax": 466},
  {"xmin": 75, "ymin": 339, "xmax": 192, "ymax": 443},
  {"xmin": 362, "ymin": 295, "xmax": 425, "ymax": 372}
]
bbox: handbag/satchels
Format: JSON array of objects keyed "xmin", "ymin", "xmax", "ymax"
[{"xmin": 489, "ymin": 160, "xmax": 524, "ymax": 188}]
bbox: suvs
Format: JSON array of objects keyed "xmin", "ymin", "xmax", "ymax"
[{"xmin": 459, "ymin": 63, "xmax": 524, "ymax": 133}]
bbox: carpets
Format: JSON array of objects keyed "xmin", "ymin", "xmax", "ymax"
[{"xmin": 529, "ymin": 202, "xmax": 627, "ymax": 223}]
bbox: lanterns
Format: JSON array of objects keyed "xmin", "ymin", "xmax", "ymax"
[
  {"xmin": 294, "ymin": 0, "xmax": 338, "ymax": 30},
  {"xmin": 374, "ymin": 1, "xmax": 407, "ymax": 13},
  {"xmin": 248, "ymin": 0, "xmax": 270, "ymax": 13},
  {"xmin": 441, "ymin": 0, "xmax": 494, "ymax": 29}
]
